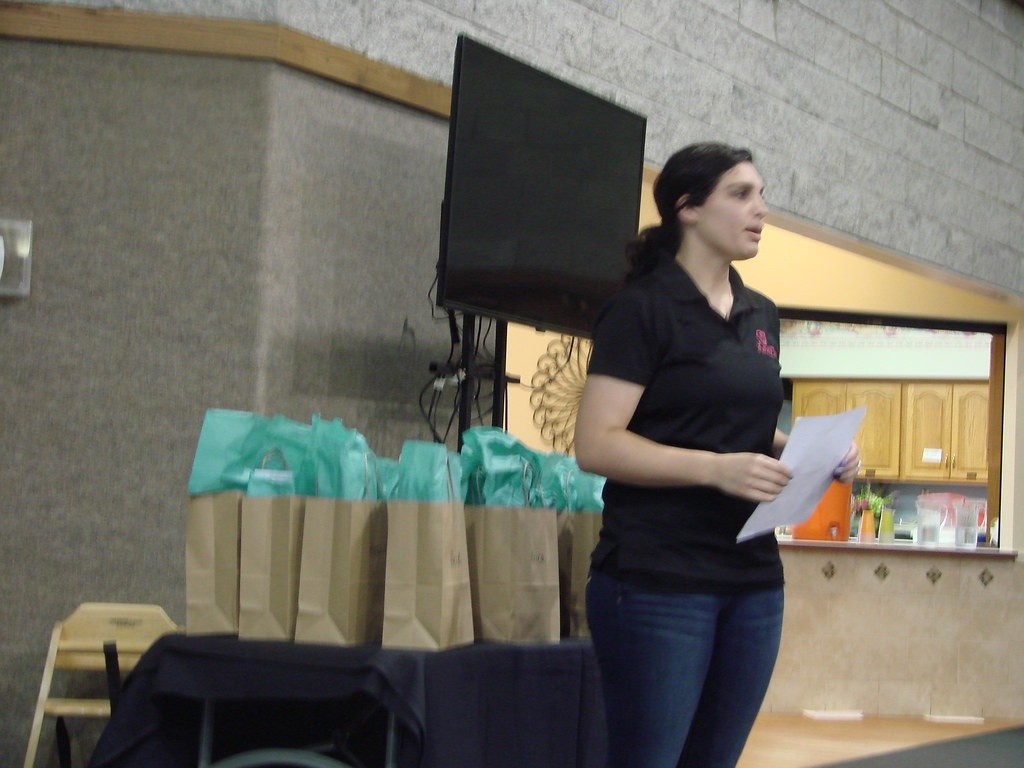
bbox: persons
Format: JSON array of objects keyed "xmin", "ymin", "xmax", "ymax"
[{"xmin": 574, "ymin": 141, "xmax": 861, "ymax": 768}]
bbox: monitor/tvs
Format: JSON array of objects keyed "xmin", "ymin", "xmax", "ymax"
[{"xmin": 437, "ymin": 31, "xmax": 647, "ymax": 339}]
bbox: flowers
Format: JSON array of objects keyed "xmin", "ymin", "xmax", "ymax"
[{"xmin": 852, "ymin": 481, "xmax": 902, "ymax": 519}]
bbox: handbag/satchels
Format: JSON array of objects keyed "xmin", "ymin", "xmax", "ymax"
[{"xmin": 186, "ymin": 448, "xmax": 603, "ymax": 652}]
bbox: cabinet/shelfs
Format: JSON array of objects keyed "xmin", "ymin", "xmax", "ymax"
[{"xmin": 791, "ymin": 381, "xmax": 989, "ymax": 484}]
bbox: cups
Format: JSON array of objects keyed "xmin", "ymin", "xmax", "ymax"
[{"xmin": 857, "ymin": 508, "xmax": 894, "ymax": 542}]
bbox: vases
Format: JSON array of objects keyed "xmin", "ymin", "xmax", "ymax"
[{"xmin": 852, "ymin": 515, "xmax": 879, "ymax": 536}]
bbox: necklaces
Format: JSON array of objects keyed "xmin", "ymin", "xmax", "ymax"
[{"xmin": 724, "ymin": 282, "xmax": 731, "ymax": 319}]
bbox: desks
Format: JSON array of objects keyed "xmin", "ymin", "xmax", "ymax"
[{"xmin": 89, "ymin": 633, "xmax": 588, "ymax": 768}]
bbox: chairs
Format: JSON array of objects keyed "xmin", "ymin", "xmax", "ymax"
[{"xmin": 22, "ymin": 605, "xmax": 179, "ymax": 768}]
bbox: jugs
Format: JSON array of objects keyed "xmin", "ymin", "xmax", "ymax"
[
  {"xmin": 913, "ymin": 494, "xmax": 967, "ymax": 547},
  {"xmin": 954, "ymin": 503, "xmax": 984, "ymax": 549},
  {"xmin": 914, "ymin": 501, "xmax": 948, "ymax": 547}
]
